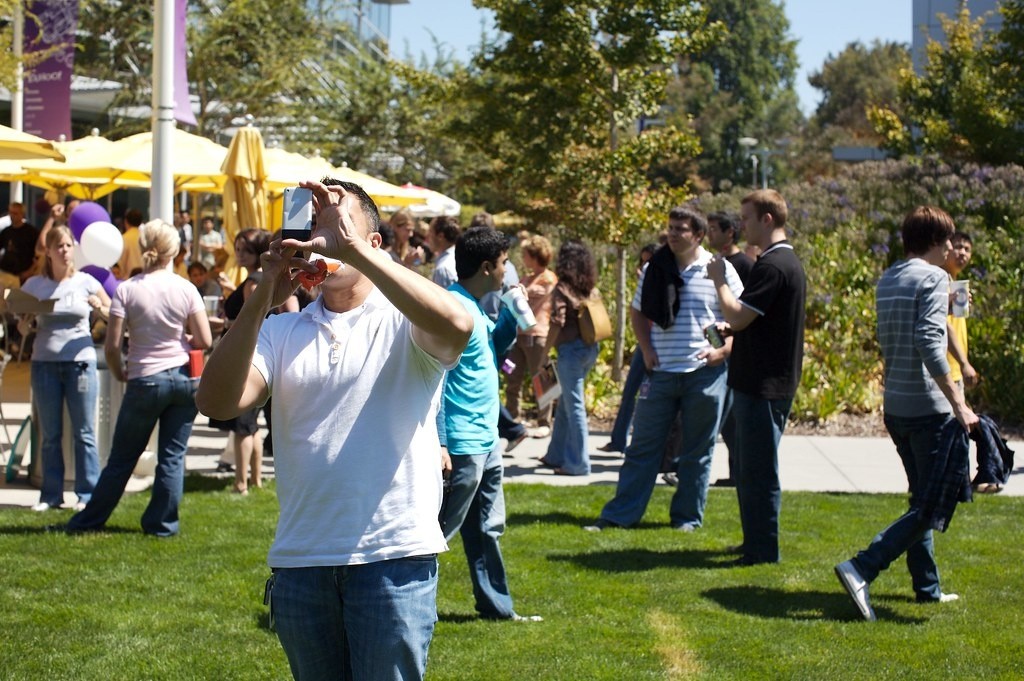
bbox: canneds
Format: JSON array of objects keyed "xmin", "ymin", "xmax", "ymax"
[{"xmin": 705, "ymin": 323, "xmax": 725, "ymax": 348}]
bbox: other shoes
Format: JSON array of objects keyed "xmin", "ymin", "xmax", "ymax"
[
  {"xmin": 217, "ymin": 463, "xmax": 238, "ymax": 472},
  {"xmin": 538, "ymin": 457, "xmax": 546, "ymax": 464},
  {"xmin": 77, "ymin": 502, "xmax": 86, "ymax": 510},
  {"xmin": 598, "ymin": 444, "xmax": 616, "ymax": 452},
  {"xmin": 533, "ymin": 425, "xmax": 550, "ymax": 437},
  {"xmin": 32, "ymin": 501, "xmax": 55, "ymax": 511},
  {"xmin": 554, "ymin": 468, "xmax": 568, "ymax": 474},
  {"xmin": 678, "ymin": 523, "xmax": 693, "ymax": 531},
  {"xmin": 734, "ymin": 558, "xmax": 747, "ymax": 565},
  {"xmin": 584, "ymin": 519, "xmax": 616, "ymax": 532},
  {"xmin": 514, "ymin": 614, "xmax": 541, "ymax": 621},
  {"xmin": 728, "ymin": 545, "xmax": 744, "ymax": 552},
  {"xmin": 505, "ymin": 433, "xmax": 526, "ymax": 451}
]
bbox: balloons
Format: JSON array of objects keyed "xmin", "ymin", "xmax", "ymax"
[{"xmin": 68, "ymin": 201, "xmax": 124, "ymax": 299}]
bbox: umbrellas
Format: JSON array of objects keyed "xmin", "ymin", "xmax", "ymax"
[
  {"xmin": 220, "ymin": 114, "xmax": 271, "ymax": 298},
  {"xmin": 0, "ymin": 122, "xmax": 461, "ymax": 217}
]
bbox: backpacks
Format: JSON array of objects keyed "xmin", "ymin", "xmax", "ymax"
[{"xmin": 573, "ymin": 287, "xmax": 612, "ymax": 343}]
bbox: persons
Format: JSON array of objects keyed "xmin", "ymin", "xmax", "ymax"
[
  {"xmin": 222, "ymin": 228, "xmax": 300, "ymax": 495},
  {"xmin": 117, "ymin": 209, "xmax": 145, "ymax": 278},
  {"xmin": 0, "ymin": 201, "xmax": 41, "ymax": 275},
  {"xmin": 428, "ymin": 216, "xmax": 461, "ymax": 287},
  {"xmin": 195, "ymin": 176, "xmax": 474, "ymax": 681},
  {"xmin": 504, "ymin": 235, "xmax": 554, "ymax": 438},
  {"xmin": 706, "ymin": 189, "xmax": 806, "ymax": 566},
  {"xmin": 596, "ymin": 244, "xmax": 659, "ymax": 458},
  {"xmin": 832, "ymin": 206, "xmax": 982, "ymax": 623},
  {"xmin": 173, "ymin": 211, "xmax": 229, "ymax": 296},
  {"xmin": 17, "ymin": 225, "xmax": 111, "ymax": 513},
  {"xmin": 537, "ymin": 239, "xmax": 601, "ymax": 476},
  {"xmin": 706, "ymin": 210, "xmax": 755, "ymax": 486},
  {"xmin": 435, "ymin": 228, "xmax": 543, "ymax": 622},
  {"xmin": 68, "ymin": 220, "xmax": 213, "ymax": 536},
  {"xmin": 378, "ymin": 209, "xmax": 430, "ymax": 269},
  {"xmin": 471, "ymin": 212, "xmax": 529, "ymax": 452},
  {"xmin": 583, "ymin": 206, "xmax": 745, "ymax": 532},
  {"xmin": 942, "ymin": 232, "xmax": 1003, "ymax": 495}
]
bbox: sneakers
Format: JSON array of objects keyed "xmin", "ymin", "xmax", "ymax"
[
  {"xmin": 834, "ymin": 560, "xmax": 875, "ymax": 620},
  {"xmin": 940, "ymin": 591, "xmax": 958, "ymax": 602}
]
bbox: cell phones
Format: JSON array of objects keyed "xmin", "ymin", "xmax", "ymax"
[{"xmin": 282, "ymin": 187, "xmax": 312, "ymax": 242}]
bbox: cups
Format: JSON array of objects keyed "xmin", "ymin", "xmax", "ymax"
[
  {"xmin": 502, "ymin": 359, "xmax": 517, "ymax": 375},
  {"xmin": 949, "ymin": 279, "xmax": 970, "ymax": 319},
  {"xmin": 203, "ymin": 295, "xmax": 218, "ymax": 317},
  {"xmin": 502, "ymin": 287, "xmax": 537, "ymax": 331}
]
